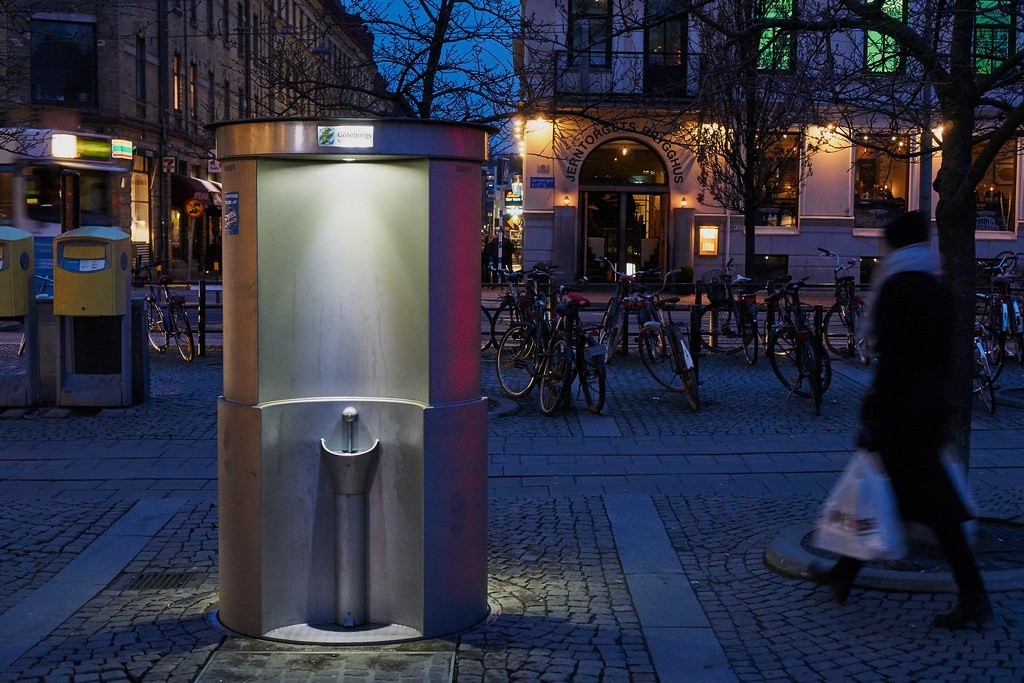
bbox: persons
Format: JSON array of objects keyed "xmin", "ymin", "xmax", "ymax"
[
  {"xmin": 494, "ymin": 227, "xmax": 516, "ymax": 290},
  {"xmin": 810, "ymin": 210, "xmax": 996, "ymax": 630},
  {"xmin": 984, "ymin": 182, "xmax": 1008, "ymax": 229},
  {"xmin": 855, "ymin": 179, "xmax": 872, "ymax": 198},
  {"xmin": 480, "ymin": 234, "xmax": 495, "ymax": 282}
]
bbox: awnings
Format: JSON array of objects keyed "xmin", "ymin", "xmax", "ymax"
[{"xmin": 169, "ymin": 171, "xmax": 223, "ymax": 213}]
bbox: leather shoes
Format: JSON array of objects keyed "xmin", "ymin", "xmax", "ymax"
[
  {"xmin": 807, "ymin": 557, "xmax": 852, "ymax": 608},
  {"xmin": 932, "ymin": 581, "xmax": 996, "ymax": 630}
]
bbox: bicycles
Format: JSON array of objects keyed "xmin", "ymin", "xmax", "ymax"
[
  {"xmin": 969, "ymin": 247, "xmax": 1024, "ymax": 413},
  {"xmin": 129, "ymin": 259, "xmax": 196, "ymax": 363},
  {"xmin": 479, "ymin": 249, "xmax": 876, "ymax": 418}
]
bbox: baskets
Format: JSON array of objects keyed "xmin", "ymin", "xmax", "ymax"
[
  {"xmin": 701, "ymin": 268, "xmax": 726, "ymax": 303},
  {"xmin": 622, "ymin": 295, "xmax": 644, "ymax": 312}
]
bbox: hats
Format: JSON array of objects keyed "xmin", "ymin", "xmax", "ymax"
[{"xmin": 883, "ymin": 210, "xmax": 926, "ymax": 249}]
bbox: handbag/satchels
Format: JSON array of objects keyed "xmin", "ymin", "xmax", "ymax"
[
  {"xmin": 938, "ymin": 443, "xmax": 985, "ymax": 536},
  {"xmin": 809, "ymin": 446, "xmax": 908, "ymax": 562}
]
